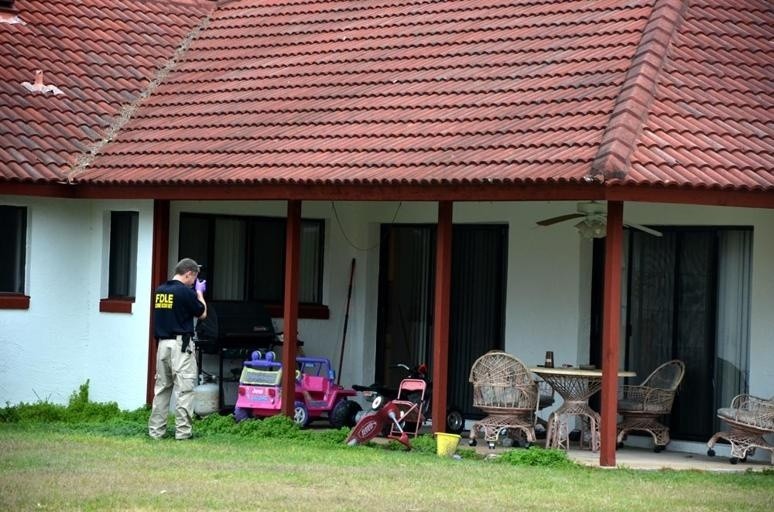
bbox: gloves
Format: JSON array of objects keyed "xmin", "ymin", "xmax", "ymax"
[{"xmin": 195, "ymin": 278, "xmax": 206, "ymax": 291}]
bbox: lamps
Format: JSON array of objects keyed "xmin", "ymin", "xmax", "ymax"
[{"xmin": 575, "ymin": 222, "xmax": 608, "ymax": 243}]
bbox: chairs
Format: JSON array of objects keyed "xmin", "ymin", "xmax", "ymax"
[
  {"xmin": 707, "ymin": 390, "xmax": 773, "ymax": 464},
  {"xmin": 467, "ymin": 349, "xmax": 540, "ymax": 450},
  {"xmin": 617, "ymin": 359, "xmax": 686, "ymax": 452}
]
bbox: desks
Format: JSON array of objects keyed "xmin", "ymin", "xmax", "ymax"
[{"xmin": 530, "ymin": 362, "xmax": 636, "ymax": 451}]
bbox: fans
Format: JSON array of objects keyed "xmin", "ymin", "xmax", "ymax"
[{"xmin": 537, "ymin": 204, "xmax": 664, "ymax": 237}]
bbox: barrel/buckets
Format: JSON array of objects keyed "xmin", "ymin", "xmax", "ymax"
[{"xmin": 434, "ymin": 432, "xmax": 462, "ymax": 456}]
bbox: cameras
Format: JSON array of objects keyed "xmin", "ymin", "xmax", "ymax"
[{"xmin": 192, "ymin": 278, "xmax": 204, "ymax": 291}]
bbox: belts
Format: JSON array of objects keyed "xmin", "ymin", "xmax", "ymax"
[{"xmin": 159, "ymin": 335, "xmax": 193, "ymax": 340}]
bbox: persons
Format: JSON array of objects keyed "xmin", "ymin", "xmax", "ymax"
[{"xmin": 146, "ymin": 257, "xmax": 207, "ymax": 440}]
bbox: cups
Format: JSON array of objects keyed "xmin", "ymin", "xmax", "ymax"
[{"xmin": 545, "ymin": 351, "xmax": 555, "ymax": 368}]
